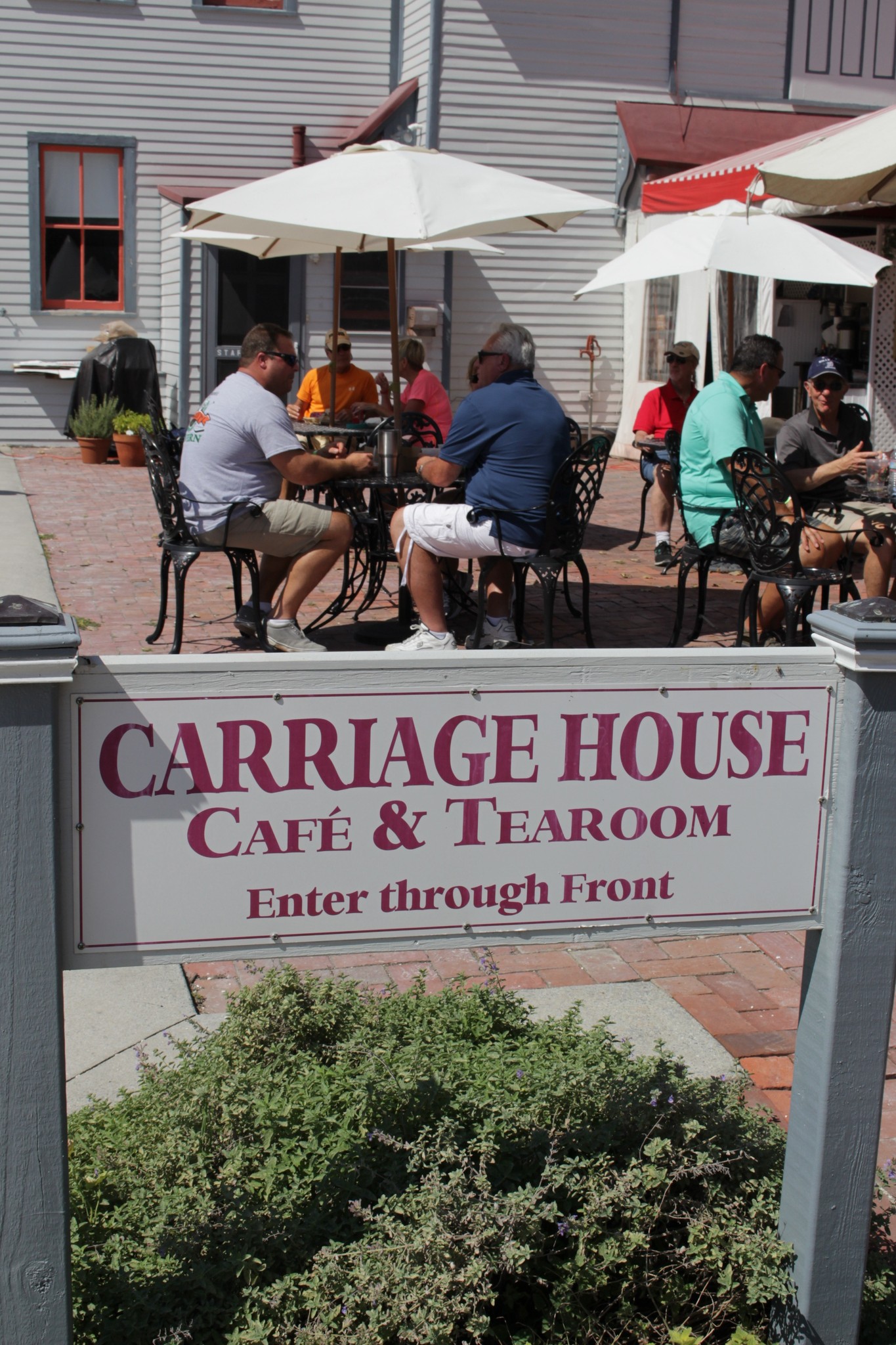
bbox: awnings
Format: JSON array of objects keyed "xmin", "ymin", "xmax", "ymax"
[
  {"xmin": 337, "ymin": 76, "xmax": 418, "ymax": 148},
  {"xmin": 614, "ymin": 103, "xmax": 859, "ymax": 214}
]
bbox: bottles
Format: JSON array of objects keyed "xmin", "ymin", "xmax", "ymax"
[{"xmin": 888, "ymin": 445, "xmax": 896, "ymax": 497}]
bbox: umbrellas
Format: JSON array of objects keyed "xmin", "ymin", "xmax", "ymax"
[
  {"xmin": 745, "ymin": 109, "xmax": 896, "ymax": 206},
  {"xmin": 181, "ymin": 140, "xmax": 626, "ymax": 477},
  {"xmin": 574, "ymin": 201, "xmax": 890, "ymax": 369},
  {"xmin": 170, "ymin": 225, "xmax": 504, "ymax": 442}
]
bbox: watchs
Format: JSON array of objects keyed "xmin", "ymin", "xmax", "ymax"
[{"xmin": 418, "ymin": 461, "xmax": 433, "ymax": 477}]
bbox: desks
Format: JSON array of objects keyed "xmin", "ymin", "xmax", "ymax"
[
  {"xmin": 849, "ymin": 486, "xmax": 896, "ymax": 503},
  {"xmin": 292, "ymin": 421, "xmax": 374, "ymax": 455},
  {"xmin": 302, "ymin": 474, "xmax": 478, "ymax": 635}
]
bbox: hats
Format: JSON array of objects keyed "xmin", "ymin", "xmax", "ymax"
[
  {"xmin": 807, "ymin": 355, "xmax": 847, "ymax": 383},
  {"xmin": 665, "ymin": 341, "xmax": 699, "ymax": 363},
  {"xmin": 325, "ymin": 327, "xmax": 351, "ymax": 348}
]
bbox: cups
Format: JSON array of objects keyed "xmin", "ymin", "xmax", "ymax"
[
  {"xmin": 866, "ymin": 458, "xmax": 888, "ymax": 491},
  {"xmin": 421, "ymin": 444, "xmax": 444, "ymax": 457},
  {"xmin": 377, "ymin": 428, "xmax": 399, "ymax": 477}
]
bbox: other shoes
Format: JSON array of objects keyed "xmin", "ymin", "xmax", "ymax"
[{"xmin": 734, "ymin": 625, "xmax": 786, "ymax": 646}]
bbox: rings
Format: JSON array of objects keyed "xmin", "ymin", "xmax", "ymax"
[{"xmin": 358, "ymin": 406, "xmax": 360, "ymax": 409}]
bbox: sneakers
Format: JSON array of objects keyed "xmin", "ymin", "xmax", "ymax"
[
  {"xmin": 385, "ymin": 622, "xmax": 458, "ymax": 651},
  {"xmin": 233, "ymin": 604, "xmax": 269, "ymax": 635},
  {"xmin": 254, "ymin": 616, "xmax": 327, "ymax": 653},
  {"xmin": 465, "ymin": 615, "xmax": 518, "ymax": 649},
  {"xmin": 654, "ymin": 540, "xmax": 672, "ymax": 566}
]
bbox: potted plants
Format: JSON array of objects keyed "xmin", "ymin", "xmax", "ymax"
[{"xmin": 69, "ymin": 393, "xmax": 164, "ymax": 467}]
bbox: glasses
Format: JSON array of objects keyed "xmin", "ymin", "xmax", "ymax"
[
  {"xmin": 666, "ymin": 354, "xmax": 687, "ymax": 364},
  {"xmin": 327, "ymin": 343, "xmax": 350, "ymax": 352},
  {"xmin": 262, "ymin": 350, "xmax": 296, "ymax": 367},
  {"xmin": 477, "ymin": 351, "xmax": 512, "ymax": 365},
  {"xmin": 469, "ymin": 375, "xmax": 479, "ymax": 384},
  {"xmin": 812, "ymin": 380, "xmax": 842, "ymax": 393},
  {"xmin": 755, "ymin": 361, "xmax": 785, "ymax": 380}
]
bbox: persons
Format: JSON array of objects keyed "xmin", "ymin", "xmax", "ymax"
[
  {"xmin": 633, "ymin": 343, "xmax": 700, "ymax": 565},
  {"xmin": 279, "ymin": 328, "xmax": 378, "ymax": 501},
  {"xmin": 679, "ymin": 334, "xmax": 843, "ymax": 646},
  {"xmin": 178, "ymin": 324, "xmax": 375, "ymax": 651},
  {"xmin": 384, "ymin": 324, "xmax": 578, "ymax": 650},
  {"xmin": 775, "ymin": 356, "xmax": 896, "ymax": 601},
  {"xmin": 439, "ymin": 355, "xmax": 482, "ymax": 625},
  {"xmin": 350, "ymin": 338, "xmax": 453, "ymax": 518}
]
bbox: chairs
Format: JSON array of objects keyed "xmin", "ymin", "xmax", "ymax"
[
  {"xmin": 140, "ymin": 411, "xmax": 616, "ymax": 654},
  {"xmin": 628, "ymin": 446, "xmax": 862, "ymax": 647}
]
